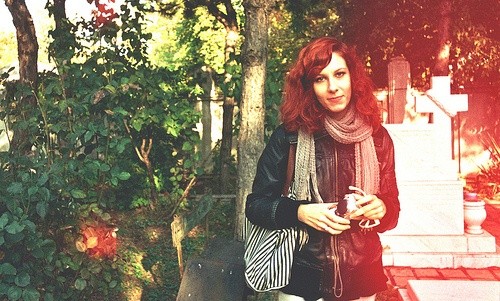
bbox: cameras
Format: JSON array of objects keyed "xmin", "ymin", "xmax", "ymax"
[{"xmin": 330, "ymin": 193, "xmax": 363, "ymax": 220}]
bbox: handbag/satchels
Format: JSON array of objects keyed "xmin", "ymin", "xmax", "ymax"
[{"xmin": 244, "ymin": 119, "xmax": 299, "ymax": 293}]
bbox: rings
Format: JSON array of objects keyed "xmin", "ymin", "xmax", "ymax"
[{"xmin": 324, "ymin": 227, "xmax": 328, "ymax": 231}]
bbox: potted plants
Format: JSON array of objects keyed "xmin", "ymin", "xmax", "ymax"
[{"xmin": 463, "ymin": 187, "xmax": 486, "ymax": 234}]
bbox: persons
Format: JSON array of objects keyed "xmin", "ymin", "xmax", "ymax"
[{"xmin": 244, "ymin": 37, "xmax": 400, "ymax": 301}]
